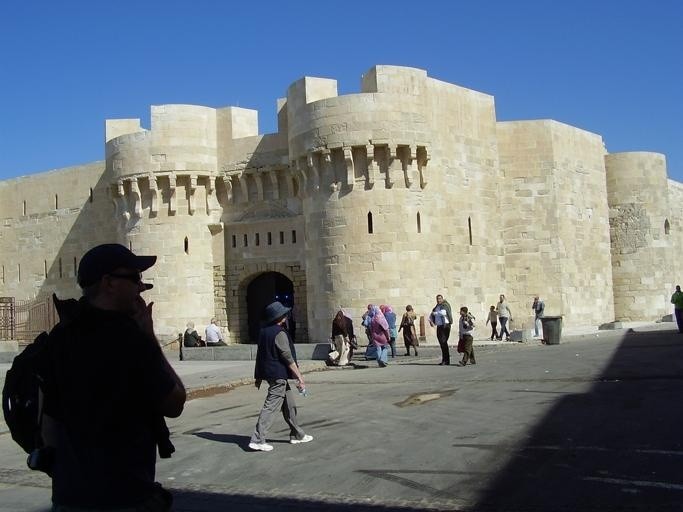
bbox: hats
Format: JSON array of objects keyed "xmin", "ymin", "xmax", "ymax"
[
  {"xmin": 263, "ymin": 300, "xmax": 292, "ymax": 327},
  {"xmin": 78, "ymin": 243, "xmax": 157, "ymax": 287}
]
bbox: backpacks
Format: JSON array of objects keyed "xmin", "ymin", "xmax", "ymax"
[{"xmin": 0, "ymin": 330, "xmax": 52, "ymax": 450}]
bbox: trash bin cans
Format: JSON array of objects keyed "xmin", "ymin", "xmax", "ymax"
[{"xmin": 539, "ymin": 316, "xmax": 562, "ymax": 345}]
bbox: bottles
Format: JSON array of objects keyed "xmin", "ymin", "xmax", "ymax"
[{"xmin": 296, "ymin": 381, "xmax": 306, "ymax": 397}]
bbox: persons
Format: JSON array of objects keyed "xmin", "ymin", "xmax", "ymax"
[
  {"xmin": 184, "ymin": 320, "xmax": 206, "ymax": 347},
  {"xmin": 496, "ymin": 295, "xmax": 513, "ymax": 342},
  {"xmin": 671, "ymin": 285, "xmax": 683, "ymax": 334},
  {"xmin": 532, "ymin": 297, "xmax": 545, "ymax": 337},
  {"xmin": 205, "ymin": 318, "xmax": 227, "ymax": 345},
  {"xmin": 398, "ymin": 305, "xmax": 419, "ymax": 356},
  {"xmin": 248, "ymin": 301, "xmax": 314, "ymax": 452},
  {"xmin": 428, "ymin": 295, "xmax": 453, "ymax": 365},
  {"xmin": 457, "ymin": 307, "xmax": 476, "ymax": 366},
  {"xmin": 486, "ymin": 306, "xmax": 498, "ymax": 341},
  {"xmin": 330, "ymin": 302, "xmax": 397, "ymax": 368},
  {"xmin": 3, "ymin": 243, "xmax": 187, "ymax": 512}
]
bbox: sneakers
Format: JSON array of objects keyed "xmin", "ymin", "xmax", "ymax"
[
  {"xmin": 248, "ymin": 440, "xmax": 273, "ymax": 453},
  {"xmin": 290, "ymin": 436, "xmax": 315, "ymax": 445},
  {"xmin": 336, "ymin": 354, "xmax": 477, "ymax": 367},
  {"xmin": 490, "ymin": 334, "xmax": 540, "ymax": 340}
]
